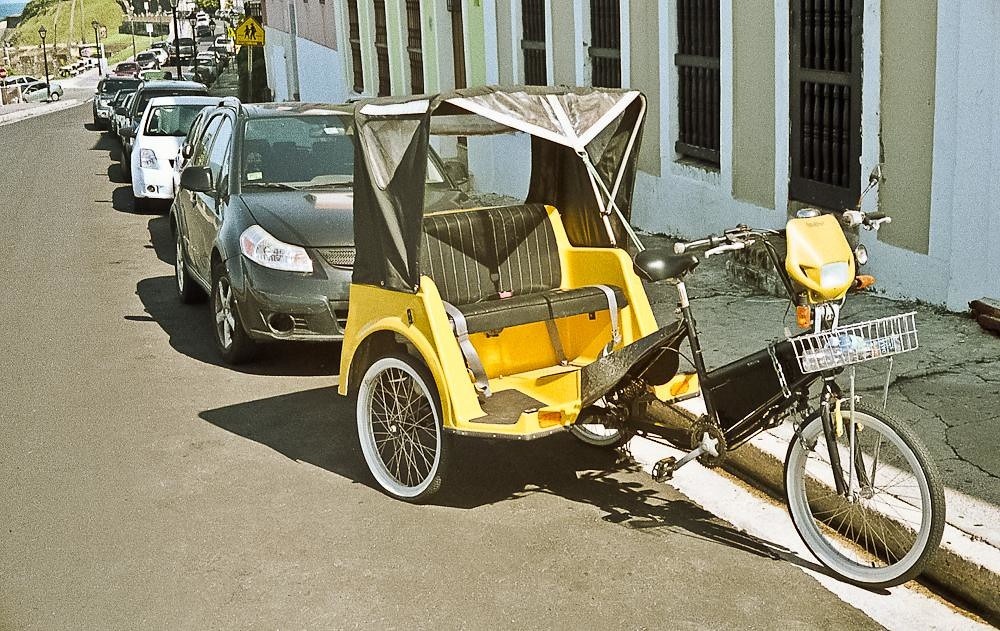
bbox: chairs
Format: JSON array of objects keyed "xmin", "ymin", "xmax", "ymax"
[
  {"xmin": 240, "ymin": 139, "xmax": 279, "ymax": 184},
  {"xmin": 332, "ymin": 134, "xmax": 358, "ymax": 176}
]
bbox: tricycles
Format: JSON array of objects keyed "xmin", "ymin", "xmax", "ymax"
[{"xmin": 337, "ymin": 82, "xmax": 947, "ymax": 590}]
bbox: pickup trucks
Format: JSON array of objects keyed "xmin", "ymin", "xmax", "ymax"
[{"xmin": 170, "ymin": 38, "xmax": 195, "ymax": 65}]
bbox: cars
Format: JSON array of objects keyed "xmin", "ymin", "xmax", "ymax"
[
  {"xmin": 0, "ymin": 75, "xmax": 41, "ymax": 93},
  {"xmin": 147, "ymin": 48, "xmax": 168, "ymax": 65},
  {"xmin": 107, "ymin": 89, "xmax": 138, "ymax": 136},
  {"xmin": 119, "ymin": 96, "xmax": 222, "ymax": 198},
  {"xmin": 196, "ymin": 9, "xmax": 239, "ymax": 37},
  {"xmin": 188, "ymin": 56, "xmax": 216, "ymax": 78},
  {"xmin": 135, "ymin": 52, "xmax": 162, "ymax": 70},
  {"xmin": 6, "ymin": 81, "xmax": 63, "ymax": 105},
  {"xmin": 151, "ymin": 41, "xmax": 170, "ymax": 54},
  {"xmin": 112, "ymin": 63, "xmax": 172, "ymax": 81}
]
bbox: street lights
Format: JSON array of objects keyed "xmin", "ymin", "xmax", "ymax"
[
  {"xmin": 38, "ymin": 25, "xmax": 52, "ymax": 102},
  {"xmin": 91, "ymin": 20, "xmax": 101, "ymax": 76},
  {"xmin": 169, "ymin": 0, "xmax": 235, "ymax": 80}
]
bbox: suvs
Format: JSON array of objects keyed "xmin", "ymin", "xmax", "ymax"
[
  {"xmin": 169, "ymin": 95, "xmax": 471, "ymax": 366},
  {"xmin": 215, "ymin": 37, "xmax": 235, "ymax": 56},
  {"xmin": 206, "ymin": 46, "xmax": 229, "ymax": 61},
  {"xmin": 197, "ymin": 51, "xmax": 223, "ymax": 72},
  {"xmin": 93, "ymin": 73, "xmax": 143, "ymax": 124},
  {"xmin": 121, "ymin": 79, "xmax": 209, "ymax": 167}
]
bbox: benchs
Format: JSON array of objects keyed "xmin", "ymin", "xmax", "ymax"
[
  {"xmin": 268, "ymin": 141, "xmax": 336, "ymax": 183},
  {"xmin": 420, "ymin": 203, "xmax": 629, "ymax": 336}
]
